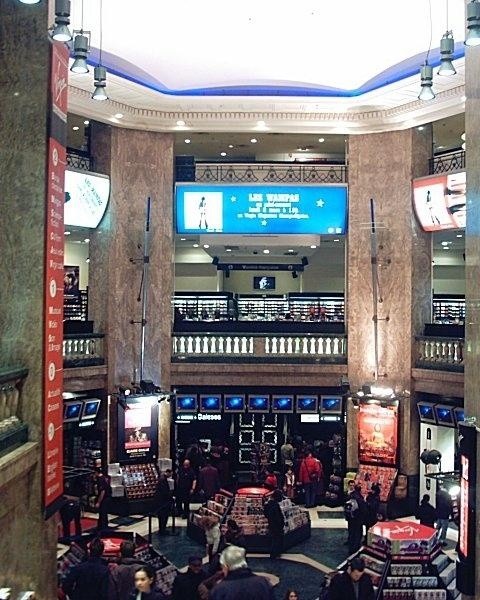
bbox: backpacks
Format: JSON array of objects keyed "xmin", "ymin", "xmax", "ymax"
[{"xmin": 344, "ymin": 499, "xmax": 359, "ymax": 521}]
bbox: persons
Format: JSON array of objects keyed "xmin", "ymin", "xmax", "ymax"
[
  {"xmin": 94, "ymin": 469, "xmax": 112, "ymax": 532},
  {"xmin": 362, "ymin": 485, "xmax": 380, "ymax": 544},
  {"xmin": 173, "ymin": 459, "xmax": 196, "ymax": 519},
  {"xmin": 281, "ymin": 438, "xmax": 297, "ymax": 471},
  {"xmin": 209, "ymin": 545, "xmax": 276, "ymax": 599},
  {"xmin": 64, "ymin": 302, "xmax": 87, "ymax": 322},
  {"xmin": 128, "ymin": 567, "xmax": 166, "ymax": 600},
  {"xmin": 213, "ymin": 441, "xmax": 231, "ymax": 488},
  {"xmin": 62, "ymin": 537, "xmax": 110, "ymax": 600},
  {"xmin": 285, "ymin": 590, "xmax": 298, "ymax": 600},
  {"xmin": 344, "ymin": 480, "xmax": 355, "ymax": 504},
  {"xmin": 196, "ymin": 457, "xmax": 220, "ymax": 501},
  {"xmin": 318, "ymin": 441, "xmax": 334, "ymax": 491},
  {"xmin": 174, "ymin": 302, "xmax": 345, "ymax": 322},
  {"xmin": 299, "ymin": 451, "xmax": 321, "ymax": 508},
  {"xmin": 108, "ymin": 541, "xmax": 147, "ymax": 600},
  {"xmin": 435, "ymin": 481, "xmax": 453, "ymax": 546},
  {"xmin": 329, "ymin": 558, "xmax": 376, "ymax": 600},
  {"xmin": 426, "ymin": 190, "xmax": 442, "ymax": 227},
  {"xmin": 415, "ymin": 495, "xmax": 437, "ymax": 528},
  {"xmin": 171, "ymin": 556, "xmax": 202, "ymax": 600},
  {"xmin": 293, "ymin": 435, "xmax": 319, "ymax": 464},
  {"xmin": 263, "ymin": 491, "xmax": 285, "ymax": 562},
  {"xmin": 312, "ymin": 457, "xmax": 325, "ymax": 504},
  {"xmin": 202, "ymin": 516, "xmax": 223, "ymax": 572},
  {"xmin": 434, "ymin": 302, "xmax": 465, "ymax": 325},
  {"xmin": 262, "ymin": 468, "xmax": 277, "ymax": 490},
  {"xmin": 154, "ymin": 469, "xmax": 171, "ymax": 532},
  {"xmin": 64, "ymin": 271, "xmax": 77, "ymax": 296},
  {"xmin": 198, "ymin": 197, "xmax": 211, "ymax": 230},
  {"xmin": 259, "ymin": 276, "xmax": 272, "ymax": 290},
  {"xmin": 225, "ymin": 519, "xmax": 247, "ymax": 549},
  {"xmin": 347, "ymin": 485, "xmax": 368, "ymax": 554},
  {"xmin": 283, "ymin": 467, "xmax": 298, "ymax": 502}
]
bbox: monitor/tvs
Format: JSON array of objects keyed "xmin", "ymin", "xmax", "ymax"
[
  {"xmin": 454, "ymin": 407, "xmax": 464, "ymax": 424},
  {"xmin": 434, "ymin": 404, "xmax": 455, "ymax": 427},
  {"xmin": 296, "ymin": 395, "xmax": 318, "ymax": 413},
  {"xmin": 200, "ymin": 394, "xmax": 221, "ymax": 412},
  {"xmin": 321, "ymin": 395, "xmax": 342, "ymax": 414},
  {"xmin": 81, "ymin": 398, "xmax": 101, "ymax": 421},
  {"xmin": 176, "ymin": 394, "xmax": 198, "ymax": 412},
  {"xmin": 417, "ymin": 401, "xmax": 436, "ymax": 424},
  {"xmin": 63, "ymin": 400, "xmax": 83, "ymax": 422},
  {"xmin": 272, "ymin": 395, "xmax": 294, "ymax": 413},
  {"xmin": 247, "ymin": 395, "xmax": 269, "ymax": 413},
  {"xmin": 224, "ymin": 394, "xmax": 246, "ymax": 412}
]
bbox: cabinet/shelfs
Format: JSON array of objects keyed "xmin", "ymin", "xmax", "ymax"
[
  {"xmin": 346, "ymin": 463, "xmax": 401, "ymax": 522},
  {"xmin": 56, "ymin": 531, "xmax": 182, "ymax": 599},
  {"xmin": 334, "ymin": 520, "xmax": 463, "ymax": 600},
  {"xmin": 186, "ymin": 482, "xmax": 312, "ymax": 555},
  {"xmin": 118, "ymin": 462, "xmax": 165, "ymax": 517}
]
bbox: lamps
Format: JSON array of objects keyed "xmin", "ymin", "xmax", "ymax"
[
  {"xmin": 69, "ymin": 35, "xmax": 90, "ymax": 75},
  {"xmin": 51, "ymin": 0, "xmax": 73, "ymax": 43},
  {"xmin": 417, "ymin": 66, "xmax": 436, "ymax": 101},
  {"xmin": 463, "ymin": 1, "xmax": 480, "ymax": 48},
  {"xmin": 91, "ymin": 66, "xmax": 109, "ymax": 101},
  {"xmin": 437, "ymin": 38, "xmax": 457, "ymax": 76}
]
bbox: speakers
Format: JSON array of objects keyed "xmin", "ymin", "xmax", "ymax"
[
  {"xmin": 140, "ymin": 380, "xmax": 154, "ymax": 394},
  {"xmin": 226, "ymin": 271, "xmax": 230, "ymax": 278},
  {"xmin": 212, "ymin": 256, "xmax": 220, "ymax": 265},
  {"xmin": 292, "ymin": 272, "xmax": 298, "ymax": 279},
  {"xmin": 362, "ymin": 385, "xmax": 371, "ymax": 395},
  {"xmin": 301, "ymin": 256, "xmax": 309, "ymax": 267},
  {"xmin": 175, "ymin": 155, "xmax": 195, "ymax": 182}
]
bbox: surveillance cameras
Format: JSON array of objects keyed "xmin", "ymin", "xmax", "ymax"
[
  {"xmin": 403, "ymin": 390, "xmax": 410, "ymax": 397},
  {"xmin": 119, "ymin": 400, "xmax": 129, "ymax": 411}
]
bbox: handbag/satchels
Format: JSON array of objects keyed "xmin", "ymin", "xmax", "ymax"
[{"xmin": 304, "ymin": 459, "xmax": 317, "ymax": 481}]
